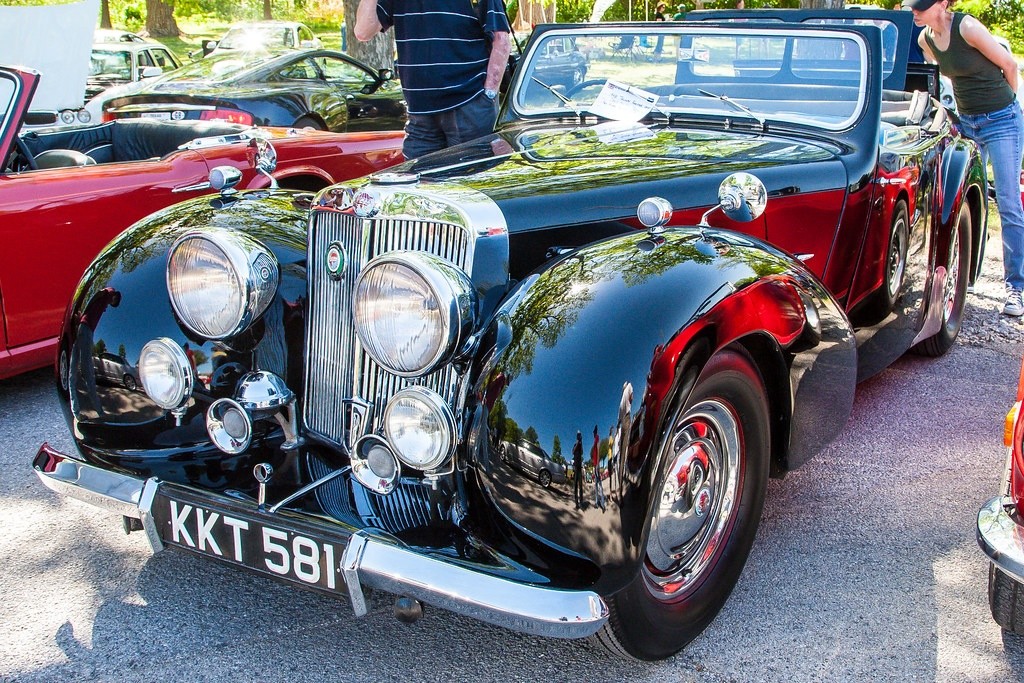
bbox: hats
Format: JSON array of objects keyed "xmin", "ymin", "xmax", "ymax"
[{"xmin": 901, "ymin": 0, "xmax": 938, "ymax": 11}]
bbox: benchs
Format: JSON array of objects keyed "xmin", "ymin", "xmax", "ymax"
[{"xmin": 111, "ymin": 118, "xmax": 246, "ymax": 162}]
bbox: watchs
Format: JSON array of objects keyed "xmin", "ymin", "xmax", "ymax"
[{"xmin": 480, "ymin": 85, "xmax": 500, "ymax": 103}]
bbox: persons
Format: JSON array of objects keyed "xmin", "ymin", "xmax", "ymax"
[
  {"xmin": 842, "ymin": 2, "xmax": 902, "ymax": 62},
  {"xmin": 902, "ymin": 0, "xmax": 1023, "ymax": 320},
  {"xmin": 654, "ymin": 1, "xmax": 666, "ymax": 62},
  {"xmin": 735, "ymin": 0, "xmax": 748, "ymax": 60},
  {"xmin": 352, "ymin": 0, "xmax": 509, "ymax": 162},
  {"xmin": 570, "ymin": 380, "xmax": 635, "ymax": 516},
  {"xmin": 672, "ymin": 3, "xmax": 688, "ymax": 64},
  {"xmin": 760, "ymin": 4, "xmax": 771, "ymax": 59}
]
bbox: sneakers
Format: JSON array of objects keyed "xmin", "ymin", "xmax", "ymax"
[{"xmin": 1003, "ymin": 286, "xmax": 1024, "ymax": 316}]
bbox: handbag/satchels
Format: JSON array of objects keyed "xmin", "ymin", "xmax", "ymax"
[{"xmin": 499, "ymin": 55, "xmax": 523, "ymax": 95}]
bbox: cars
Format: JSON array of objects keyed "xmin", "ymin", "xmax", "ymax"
[
  {"xmin": 29, "ymin": 8, "xmax": 991, "ymax": 664},
  {"xmin": 499, "ymin": 31, "xmax": 590, "ymax": 94},
  {"xmin": 0, "ymin": 64, "xmax": 406, "ymax": 381},
  {"xmin": 204, "ymin": 21, "xmax": 323, "ymax": 59},
  {"xmin": 0, "ymin": 0, "xmax": 184, "ymax": 136},
  {"xmin": 101, "ymin": 50, "xmax": 407, "ymax": 132}
]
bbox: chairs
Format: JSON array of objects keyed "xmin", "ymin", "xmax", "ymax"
[{"xmin": 22, "ymin": 149, "xmax": 98, "ymax": 173}]
